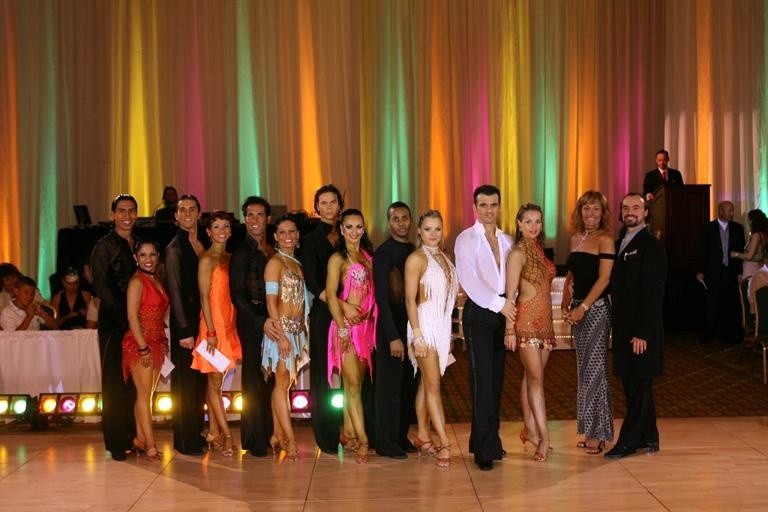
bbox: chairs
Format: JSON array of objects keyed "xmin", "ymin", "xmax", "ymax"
[
  {"xmin": 39, "ymin": 305, "xmax": 57, "ymax": 331},
  {"xmin": 733, "ymin": 275, "xmax": 757, "ymax": 365},
  {"xmin": 749, "ymin": 285, "xmax": 768, "ymax": 385},
  {"xmin": 48, "ymin": 273, "xmax": 95, "ymax": 301}
]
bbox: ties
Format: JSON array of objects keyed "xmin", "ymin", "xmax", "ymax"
[{"xmin": 660, "ymin": 170, "xmax": 667, "ymax": 180}]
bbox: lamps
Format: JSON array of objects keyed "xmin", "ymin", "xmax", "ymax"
[
  {"xmin": 96, "ymin": 392, "xmax": 103, "ymax": 417},
  {"xmin": 326, "ymin": 390, "xmax": 346, "ymax": 415},
  {"xmin": 222, "ymin": 391, "xmax": 234, "ymax": 413},
  {"xmin": 290, "ymin": 390, "xmax": 312, "ymax": 413},
  {"xmin": 57, "ymin": 394, "xmax": 78, "ymax": 422},
  {"xmin": 38, "ymin": 392, "xmax": 59, "ymax": 420},
  {"xmin": 78, "ymin": 392, "xmax": 98, "ymax": 416},
  {"xmin": 232, "ymin": 392, "xmax": 246, "ymax": 413},
  {"xmin": 12, "ymin": 394, "xmax": 35, "ymax": 422},
  {"xmin": 0, "ymin": 396, "xmax": 10, "ymax": 419},
  {"xmin": 152, "ymin": 391, "xmax": 173, "ymax": 417}
]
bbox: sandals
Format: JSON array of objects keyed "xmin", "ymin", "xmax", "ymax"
[
  {"xmin": 586, "ymin": 434, "xmax": 605, "ymax": 455},
  {"xmin": 576, "ymin": 432, "xmax": 589, "ymax": 447}
]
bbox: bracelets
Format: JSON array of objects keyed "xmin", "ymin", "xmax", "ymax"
[
  {"xmin": 206, "ymin": 329, "xmax": 217, "ymax": 337},
  {"xmin": 579, "ymin": 302, "xmax": 590, "ymax": 312},
  {"xmin": 336, "ymin": 329, "xmax": 349, "ymax": 338},
  {"xmin": 735, "ymin": 252, "xmax": 738, "ymax": 259},
  {"xmin": 137, "ymin": 345, "xmax": 151, "ymax": 357},
  {"xmin": 505, "ymin": 327, "xmax": 515, "ymax": 336},
  {"xmin": 413, "ymin": 335, "xmax": 425, "ymax": 346}
]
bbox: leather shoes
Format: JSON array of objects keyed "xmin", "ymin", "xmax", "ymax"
[
  {"xmin": 375, "ymin": 444, "xmax": 409, "ymax": 460},
  {"xmin": 469, "ymin": 438, "xmax": 507, "ymax": 458},
  {"xmin": 109, "ymin": 447, "xmax": 127, "ymax": 461},
  {"xmin": 251, "ymin": 443, "xmax": 268, "ymax": 456},
  {"xmin": 604, "ymin": 444, "xmax": 638, "ymax": 459},
  {"xmin": 477, "ymin": 458, "xmax": 493, "ymax": 470},
  {"xmin": 184, "ymin": 445, "xmax": 205, "ymax": 457},
  {"xmin": 321, "ymin": 439, "xmax": 337, "ymax": 455},
  {"xmin": 639, "ymin": 442, "xmax": 660, "ymax": 452},
  {"xmin": 396, "ymin": 435, "xmax": 416, "ymax": 453}
]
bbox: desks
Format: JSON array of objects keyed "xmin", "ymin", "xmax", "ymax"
[{"xmin": 0, "ymin": 331, "xmax": 344, "ymax": 393}]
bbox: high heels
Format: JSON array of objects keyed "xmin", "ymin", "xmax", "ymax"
[
  {"xmin": 206, "ymin": 432, "xmax": 224, "ymax": 451},
  {"xmin": 436, "ymin": 443, "xmax": 452, "ymax": 468},
  {"xmin": 222, "ymin": 434, "xmax": 234, "ymax": 459},
  {"xmin": 144, "ymin": 444, "xmax": 160, "ymax": 463},
  {"xmin": 534, "ymin": 434, "xmax": 550, "ymax": 461},
  {"xmin": 284, "ymin": 439, "xmax": 299, "ymax": 463},
  {"xmin": 134, "ymin": 439, "xmax": 150, "ymax": 456},
  {"xmin": 353, "ymin": 440, "xmax": 370, "ymax": 464},
  {"xmin": 339, "ymin": 433, "xmax": 360, "ymax": 454},
  {"xmin": 520, "ymin": 431, "xmax": 554, "ymax": 454},
  {"xmin": 269, "ymin": 434, "xmax": 286, "ymax": 453},
  {"xmin": 413, "ymin": 435, "xmax": 438, "ymax": 457}
]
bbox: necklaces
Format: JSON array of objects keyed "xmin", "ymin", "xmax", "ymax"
[
  {"xmin": 573, "ymin": 227, "xmax": 602, "ymax": 252},
  {"xmin": 138, "ymin": 268, "xmax": 156, "ymax": 276},
  {"xmin": 484, "ymin": 230, "xmax": 497, "ymax": 250},
  {"xmin": 422, "ymin": 244, "xmax": 450, "ymax": 279},
  {"xmin": 520, "ymin": 237, "xmax": 547, "ymax": 266}
]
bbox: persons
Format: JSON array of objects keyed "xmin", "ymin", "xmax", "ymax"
[
  {"xmin": 0, "ymin": 263, "xmax": 44, "ymax": 316},
  {"xmin": 604, "ymin": 193, "xmax": 667, "ymax": 458},
  {"xmin": 373, "ymin": 202, "xmax": 421, "ymax": 459},
  {"xmin": 51, "ymin": 266, "xmax": 91, "ymax": 330},
  {"xmin": 302, "ymin": 185, "xmax": 374, "ymax": 454},
  {"xmin": 697, "ymin": 200, "xmax": 745, "ymax": 343},
  {"xmin": 405, "ymin": 210, "xmax": 460, "ymax": 468},
  {"xmin": 82, "ymin": 263, "xmax": 94, "ymax": 295},
  {"xmin": 0, "ymin": 277, "xmax": 58, "ymax": 431},
  {"xmin": 561, "ymin": 190, "xmax": 614, "ymax": 454},
  {"xmin": 166, "ymin": 195, "xmax": 212, "ymax": 456},
  {"xmin": 326, "ymin": 209, "xmax": 378, "ymax": 464},
  {"xmin": 229, "ymin": 196, "xmax": 283, "ymax": 457},
  {"xmin": 155, "ymin": 186, "xmax": 178, "ymax": 218},
  {"xmin": 748, "ymin": 245, "xmax": 768, "ymax": 329},
  {"xmin": 261, "ymin": 214, "xmax": 316, "ymax": 461},
  {"xmin": 455, "ymin": 185, "xmax": 517, "ymax": 472},
  {"xmin": 643, "ymin": 151, "xmax": 683, "ymax": 200},
  {"xmin": 504, "ymin": 203, "xmax": 556, "ymax": 462},
  {"xmin": 122, "ymin": 240, "xmax": 170, "ymax": 463},
  {"xmin": 91, "ymin": 194, "xmax": 140, "ymax": 461},
  {"xmin": 87, "ymin": 296, "xmax": 101, "ymax": 329},
  {"xmin": 190, "ymin": 210, "xmax": 242, "ymax": 456},
  {"xmin": 730, "ymin": 209, "xmax": 768, "ymax": 330}
]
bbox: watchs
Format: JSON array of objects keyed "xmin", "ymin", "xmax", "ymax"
[{"xmin": 561, "ymin": 302, "xmax": 569, "ymax": 309}]
